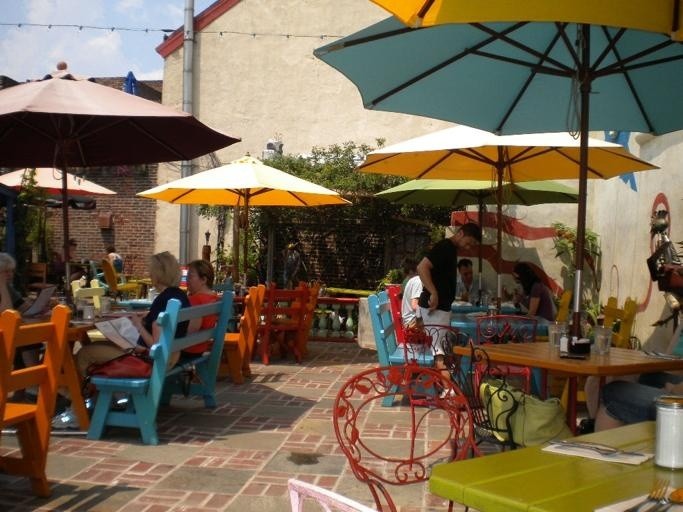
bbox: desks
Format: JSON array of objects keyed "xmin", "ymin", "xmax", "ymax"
[
  {"xmin": 451, "ymin": 339, "xmax": 682, "ymax": 437},
  {"xmin": 429, "ymin": 420, "xmax": 683, "ymax": 512}
]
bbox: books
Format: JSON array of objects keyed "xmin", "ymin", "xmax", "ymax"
[{"xmin": 93, "ymin": 317, "xmax": 140, "ymax": 351}]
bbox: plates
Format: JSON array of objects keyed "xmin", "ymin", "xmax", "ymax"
[{"xmin": 117, "ymin": 302, "xmax": 152, "ymax": 308}]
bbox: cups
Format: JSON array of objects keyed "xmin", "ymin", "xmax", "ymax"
[
  {"xmin": 549, "ymin": 321, "xmax": 569, "ymax": 351},
  {"xmin": 592, "ymin": 326, "xmax": 614, "ymax": 355},
  {"xmin": 487, "ymin": 304, "xmax": 497, "ymax": 317},
  {"xmin": 455, "ymin": 285, "xmax": 491, "ymax": 307},
  {"xmin": 101, "ymin": 298, "xmax": 112, "ymax": 314}
]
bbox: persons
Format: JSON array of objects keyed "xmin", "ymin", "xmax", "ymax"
[
  {"xmin": 404, "ymin": 266, "xmax": 428, "ymax": 332},
  {"xmin": 50, "ymin": 252, "xmax": 189, "ymax": 428},
  {"xmin": 55, "ymin": 241, "xmax": 85, "ymax": 282},
  {"xmin": 501, "ymin": 266, "xmax": 553, "ymax": 323},
  {"xmin": 103, "ymin": 246, "xmax": 122, "ymax": 272},
  {"xmin": 583, "ymin": 321, "xmax": 682, "ymax": 420},
  {"xmin": 452, "ymin": 256, "xmax": 482, "ymax": 301},
  {"xmin": 179, "ymin": 259, "xmax": 219, "ymax": 357},
  {"xmin": 0, "ymin": 256, "xmax": 51, "ymax": 436},
  {"xmin": 592, "ymin": 369, "xmax": 683, "ymax": 435},
  {"xmin": 419, "ymin": 218, "xmax": 475, "ymax": 398}
]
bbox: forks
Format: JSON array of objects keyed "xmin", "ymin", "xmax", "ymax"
[
  {"xmin": 623, "ymin": 478, "xmax": 671, "ymax": 511},
  {"xmin": 545, "ymin": 438, "xmax": 622, "ymax": 456},
  {"xmin": 642, "ymin": 349, "xmax": 681, "ymax": 359}
]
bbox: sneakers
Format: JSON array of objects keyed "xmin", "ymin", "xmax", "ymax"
[
  {"xmin": 438, "ymin": 387, "xmax": 464, "ymax": 409},
  {"xmin": 50, "ymin": 405, "xmax": 80, "ymax": 429}
]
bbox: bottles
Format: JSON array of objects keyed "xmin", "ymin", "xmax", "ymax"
[
  {"xmin": 559, "ymin": 331, "xmax": 568, "ymax": 353},
  {"xmin": 652, "ymin": 395, "xmax": 683, "ymax": 470},
  {"xmin": 55, "ymin": 296, "xmax": 94, "ymax": 322}
]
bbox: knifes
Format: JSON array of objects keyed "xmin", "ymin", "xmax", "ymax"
[{"xmin": 557, "ymin": 438, "xmax": 644, "ymax": 457}]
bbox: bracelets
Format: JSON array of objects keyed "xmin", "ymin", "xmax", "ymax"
[{"xmin": 137, "ymin": 326, "xmax": 143, "ymax": 334}]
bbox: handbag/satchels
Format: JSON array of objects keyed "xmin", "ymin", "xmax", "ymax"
[
  {"xmin": 479, "ymin": 378, "xmax": 575, "ymax": 448},
  {"xmin": 86, "ymin": 346, "xmax": 153, "ymax": 378},
  {"xmin": 657, "ymin": 260, "xmax": 683, "ymax": 292}
]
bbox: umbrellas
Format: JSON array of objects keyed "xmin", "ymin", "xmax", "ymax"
[
  {"xmin": 370, "ymin": 177, "xmax": 582, "ymax": 300},
  {"xmin": 315, "ymin": 24, "xmax": 683, "ymax": 433},
  {"xmin": 352, "ymin": 124, "xmax": 659, "ymax": 306},
  {"xmin": 0, "ymin": 166, "xmax": 119, "ymax": 202},
  {"xmin": 136, "ymin": 151, "xmax": 351, "ymax": 284},
  {"xmin": 0, "ymin": 61, "xmax": 241, "ymax": 305},
  {"xmin": 365, "ymin": 0, "xmax": 683, "ymax": 52}
]
